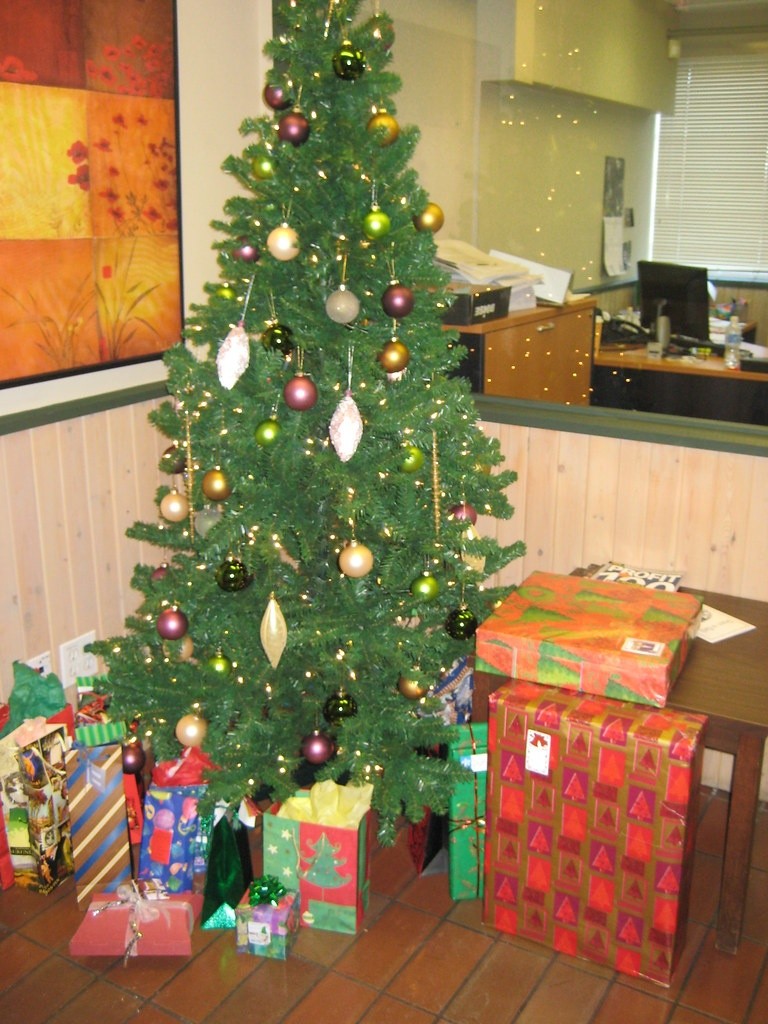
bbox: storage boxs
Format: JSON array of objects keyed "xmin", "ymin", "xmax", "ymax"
[
  {"xmin": 475, "ymin": 570, "xmax": 704, "ymax": 709},
  {"xmin": 69, "ymin": 893, "xmax": 203, "ymax": 956},
  {"xmin": 439, "ymin": 722, "xmax": 487, "ymax": 901},
  {"xmin": 441, "ymin": 285, "xmax": 512, "ymax": 325},
  {"xmin": 236, "ymin": 886, "xmax": 299, "ymax": 959},
  {"xmin": 481, "ymin": 680, "xmax": 709, "ymax": 988}
]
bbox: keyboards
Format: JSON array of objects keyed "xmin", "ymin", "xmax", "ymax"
[{"xmin": 670, "ymin": 336, "xmax": 752, "ymax": 358}]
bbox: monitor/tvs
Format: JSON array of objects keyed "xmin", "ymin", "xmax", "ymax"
[{"xmin": 638, "ymin": 260, "xmax": 710, "ymax": 343}]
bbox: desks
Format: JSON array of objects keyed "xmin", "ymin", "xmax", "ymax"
[
  {"xmin": 594, "ymin": 322, "xmax": 768, "ymax": 426},
  {"xmin": 473, "ymin": 566, "xmax": 768, "ymax": 956}
]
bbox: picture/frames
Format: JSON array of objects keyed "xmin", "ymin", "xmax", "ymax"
[{"xmin": 0, "ymin": 0, "xmax": 185, "ymax": 390}]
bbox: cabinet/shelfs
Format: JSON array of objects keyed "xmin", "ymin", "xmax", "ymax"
[{"xmin": 442, "ymin": 297, "xmax": 598, "ymax": 407}]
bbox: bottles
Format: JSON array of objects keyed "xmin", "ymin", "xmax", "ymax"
[{"xmin": 724, "ymin": 316, "xmax": 742, "ymax": 369}]
